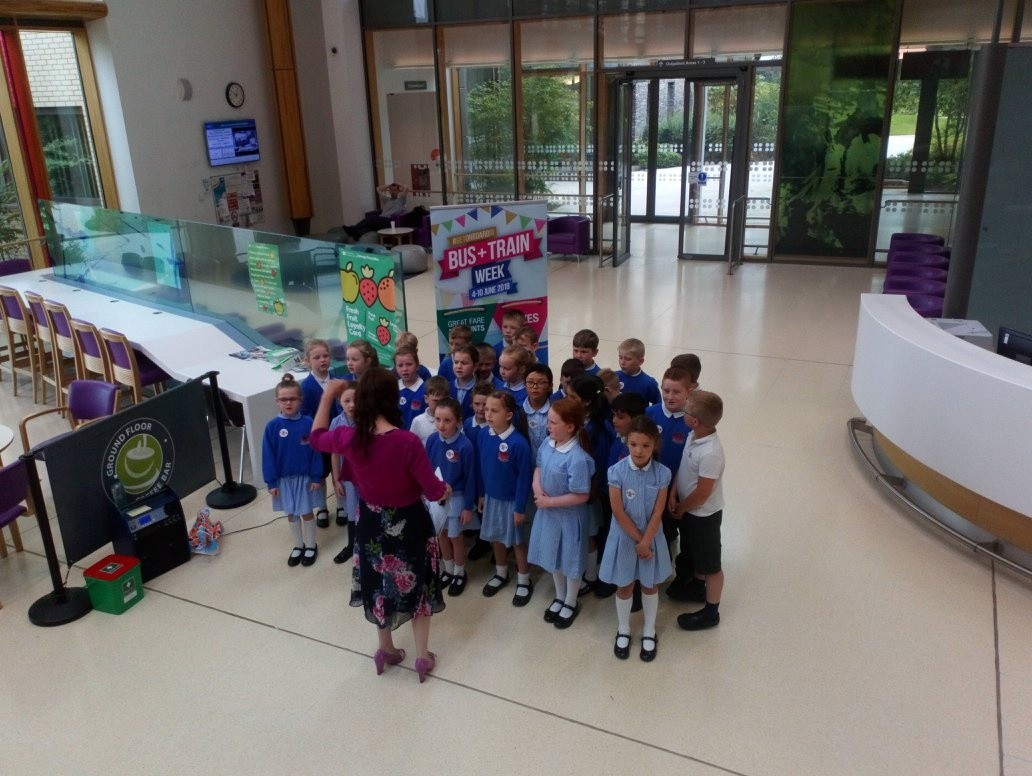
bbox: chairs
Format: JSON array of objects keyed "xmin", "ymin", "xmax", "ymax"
[
  {"xmin": 187, "ymin": 378, "xmax": 249, "ymax": 483},
  {"xmin": 882, "ymin": 233, "xmax": 951, "ymax": 318},
  {"xmin": 0, "ymin": 461, "xmax": 36, "ymax": 558},
  {"xmin": 547, "ymin": 216, "xmax": 590, "ymax": 264},
  {"xmin": 396, "ymin": 211, "xmax": 432, "ymax": 253}
]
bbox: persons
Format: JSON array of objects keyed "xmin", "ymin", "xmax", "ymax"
[
  {"xmin": 599, "ymin": 416, "xmax": 674, "ymax": 663},
  {"xmin": 410, "ymin": 308, "xmax": 702, "ymax": 465},
  {"xmin": 343, "ymin": 183, "xmax": 408, "ymax": 242},
  {"xmin": 392, "ymin": 347, "xmax": 429, "ymax": 431},
  {"xmin": 645, "ymin": 367, "xmax": 693, "ymax": 563},
  {"xmin": 422, "ymin": 397, "xmax": 474, "ymax": 597},
  {"xmin": 299, "ymin": 339, "xmax": 341, "ymax": 421},
  {"xmin": 527, "ymin": 393, "xmax": 595, "ymax": 629},
  {"xmin": 667, "ymin": 389, "xmax": 725, "ymax": 631},
  {"xmin": 474, "ymin": 391, "xmax": 533, "ymax": 607},
  {"xmin": 309, "ymin": 367, "xmax": 452, "ymax": 684},
  {"xmin": 335, "ymin": 339, "xmax": 379, "ymax": 414},
  {"xmin": 261, "ymin": 373, "xmax": 324, "ymax": 567},
  {"xmin": 329, "ymin": 381, "xmax": 360, "ymax": 563},
  {"xmin": 391, "ymin": 333, "xmax": 432, "ymax": 382},
  {"xmin": 604, "ymin": 390, "xmax": 660, "ymax": 548}
]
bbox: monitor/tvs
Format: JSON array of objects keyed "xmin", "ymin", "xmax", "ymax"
[
  {"xmin": 202, "ymin": 119, "xmax": 261, "ymax": 167},
  {"xmin": 997, "ymin": 327, "xmax": 1032, "ymax": 366}
]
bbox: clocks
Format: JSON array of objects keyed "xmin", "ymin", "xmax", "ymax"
[{"xmin": 226, "ymin": 83, "xmax": 245, "ymax": 108}]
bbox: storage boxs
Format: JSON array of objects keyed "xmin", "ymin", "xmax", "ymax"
[{"xmin": 83, "ymin": 554, "xmax": 144, "ymax": 615}]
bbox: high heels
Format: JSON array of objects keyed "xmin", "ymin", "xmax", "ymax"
[
  {"xmin": 374, "ymin": 649, "xmax": 405, "ymax": 675},
  {"xmin": 415, "ymin": 652, "xmax": 435, "ymax": 683}
]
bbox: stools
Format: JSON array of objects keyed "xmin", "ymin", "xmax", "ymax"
[
  {"xmin": 0, "ymin": 259, "xmax": 171, "ymax": 423},
  {"xmin": 303, "ymin": 226, "xmax": 427, "ymax": 273}
]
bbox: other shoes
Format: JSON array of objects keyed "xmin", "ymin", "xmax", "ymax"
[
  {"xmin": 490, "ymin": 546, "xmax": 513, "ymax": 564},
  {"xmin": 578, "ymin": 573, "xmax": 598, "ymax": 597},
  {"xmin": 544, "ymin": 599, "xmax": 564, "ymax": 623},
  {"xmin": 670, "ymin": 585, "xmax": 706, "ymax": 603},
  {"xmin": 614, "ymin": 631, "xmax": 631, "ymax": 659},
  {"xmin": 439, "ymin": 571, "xmax": 453, "ymax": 589},
  {"xmin": 631, "ymin": 580, "xmax": 642, "ymax": 612},
  {"xmin": 512, "ymin": 579, "xmax": 533, "ymax": 606},
  {"xmin": 353, "ymin": 234, "xmax": 359, "ymax": 241},
  {"xmin": 483, "ymin": 573, "xmax": 509, "ymax": 596},
  {"xmin": 343, "ymin": 226, "xmax": 352, "ymax": 237},
  {"xmin": 640, "ymin": 634, "xmax": 658, "ymax": 662},
  {"xmin": 593, "ymin": 579, "xmax": 617, "ymax": 598},
  {"xmin": 448, "ymin": 570, "xmax": 467, "ymax": 595},
  {"xmin": 288, "ymin": 507, "xmax": 353, "ymax": 566},
  {"xmin": 468, "ymin": 544, "xmax": 492, "ymax": 561},
  {"xmin": 677, "ymin": 609, "xmax": 719, "ymax": 630},
  {"xmin": 555, "ymin": 601, "xmax": 579, "ymax": 629}
]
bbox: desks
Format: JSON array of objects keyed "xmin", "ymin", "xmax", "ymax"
[
  {"xmin": 0, "ymin": 267, "xmax": 334, "ymax": 490},
  {"xmin": 0, "ymin": 424, "xmax": 14, "ymax": 467},
  {"xmin": 377, "ymin": 228, "xmax": 414, "ymax": 246}
]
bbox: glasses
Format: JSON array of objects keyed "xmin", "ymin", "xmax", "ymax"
[
  {"xmin": 277, "ymin": 397, "xmax": 300, "ymax": 402},
  {"xmin": 524, "ymin": 380, "xmax": 550, "ymax": 388},
  {"xmin": 681, "ymin": 408, "xmax": 700, "ymax": 424}
]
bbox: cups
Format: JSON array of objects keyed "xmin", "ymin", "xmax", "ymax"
[{"xmin": 390, "ymin": 221, "xmax": 395, "ymax": 229}]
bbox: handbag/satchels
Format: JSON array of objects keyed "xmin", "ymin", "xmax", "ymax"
[{"xmin": 186, "ymin": 508, "xmax": 221, "ymax": 556}]
